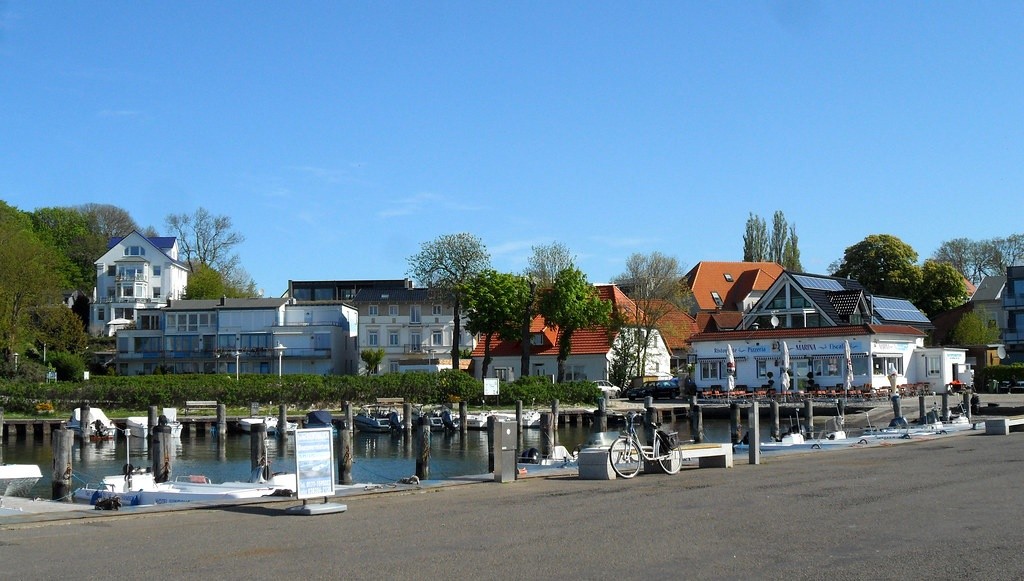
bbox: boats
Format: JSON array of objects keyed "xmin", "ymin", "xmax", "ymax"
[
  {"xmin": 353, "ymin": 403, "xmax": 413, "ymax": 433},
  {"xmin": 515, "ymin": 429, "xmax": 646, "ymax": 474},
  {"xmin": 453, "ymin": 409, "xmax": 543, "ymax": 428},
  {"xmin": 302, "ymin": 411, "xmax": 338, "ymax": 434},
  {"xmin": 733, "ymin": 396, "xmax": 986, "ymax": 454},
  {"xmin": 235, "ymin": 415, "xmax": 299, "ymax": 433},
  {"xmin": 69, "ymin": 429, "xmax": 380, "ymax": 507},
  {"xmin": 126, "ymin": 416, "xmax": 183, "ymax": 438},
  {"xmin": 64, "ymin": 407, "xmax": 120, "ymax": 441},
  {"xmin": 412, "ymin": 403, "xmax": 453, "ymax": 431},
  {"xmin": 0, "ymin": 463, "xmax": 44, "ymax": 499}
]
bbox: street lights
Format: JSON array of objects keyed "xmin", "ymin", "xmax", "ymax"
[
  {"xmin": 273, "ymin": 344, "xmax": 288, "ymax": 379},
  {"xmin": 235, "ymin": 350, "xmax": 242, "ymax": 380},
  {"xmin": 13, "ymin": 352, "xmax": 20, "ymax": 372}
]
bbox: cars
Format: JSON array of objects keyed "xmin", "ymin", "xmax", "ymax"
[
  {"xmin": 589, "ymin": 380, "xmax": 621, "ymax": 400},
  {"xmin": 627, "ymin": 380, "xmax": 682, "ymax": 401}
]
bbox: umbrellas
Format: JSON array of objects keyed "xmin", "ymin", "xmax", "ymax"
[
  {"xmin": 726, "ymin": 343, "xmax": 736, "ymax": 403},
  {"xmin": 843, "ymin": 339, "xmax": 853, "ymax": 404},
  {"xmin": 781, "ymin": 341, "xmax": 790, "ymax": 403}
]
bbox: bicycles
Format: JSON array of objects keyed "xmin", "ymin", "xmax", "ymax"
[{"xmin": 608, "ymin": 407, "xmax": 685, "ymax": 480}]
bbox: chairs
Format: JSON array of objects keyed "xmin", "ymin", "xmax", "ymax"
[{"xmin": 702, "ymin": 382, "xmax": 930, "ymax": 405}]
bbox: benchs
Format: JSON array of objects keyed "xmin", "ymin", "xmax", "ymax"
[
  {"xmin": 578, "ymin": 442, "xmax": 732, "ymax": 479},
  {"xmin": 985, "ymin": 418, "xmax": 1024, "ymax": 436}
]
